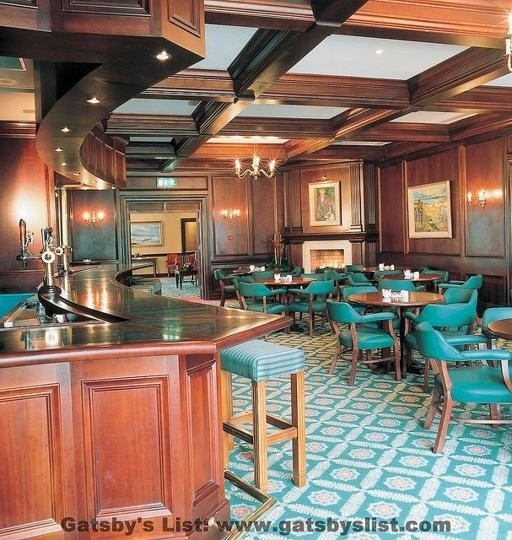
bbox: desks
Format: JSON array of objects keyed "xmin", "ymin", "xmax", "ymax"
[{"xmin": 131, "ymin": 256, "xmax": 158, "ymax": 278}]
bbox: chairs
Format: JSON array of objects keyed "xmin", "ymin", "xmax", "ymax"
[{"xmin": 166, "ymin": 251, "xmax": 200, "ymax": 290}]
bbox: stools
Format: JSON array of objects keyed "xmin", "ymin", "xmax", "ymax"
[
  {"xmin": 129, "ymin": 276, "xmax": 162, "ymax": 296},
  {"xmin": 219, "ymin": 340, "xmax": 307, "ymax": 495}
]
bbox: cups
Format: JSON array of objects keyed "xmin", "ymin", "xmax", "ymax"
[
  {"xmin": 403, "ymin": 270, "xmax": 420, "ymax": 280},
  {"xmin": 273, "ymin": 272, "xmax": 292, "ymax": 283},
  {"xmin": 383, "ymin": 298, "xmax": 410, "ymax": 304},
  {"xmin": 249, "ymin": 265, "xmax": 265, "ymax": 272},
  {"xmin": 379, "ymin": 263, "xmax": 395, "ymax": 271},
  {"xmin": 381, "ymin": 288, "xmax": 411, "ymax": 299}
]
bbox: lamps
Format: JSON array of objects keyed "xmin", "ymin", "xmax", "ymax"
[
  {"xmin": 230, "ymin": 136, "xmax": 279, "ymax": 181},
  {"xmin": 82, "ymin": 209, "xmax": 104, "ymax": 224},
  {"xmin": 467, "ymin": 188, "xmax": 485, "ymax": 208},
  {"xmin": 220, "ymin": 207, "xmax": 241, "ymax": 224},
  {"xmin": 503, "ymin": 12, "xmax": 512, "ymax": 72}
]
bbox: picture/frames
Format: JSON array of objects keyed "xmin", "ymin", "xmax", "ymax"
[
  {"xmin": 307, "ymin": 178, "xmax": 342, "ymax": 227},
  {"xmin": 407, "ymin": 179, "xmax": 453, "ymax": 239},
  {"xmin": 181, "ymin": 218, "xmax": 196, "ymax": 253},
  {"xmin": 130, "ymin": 221, "xmax": 163, "ymax": 247}
]
